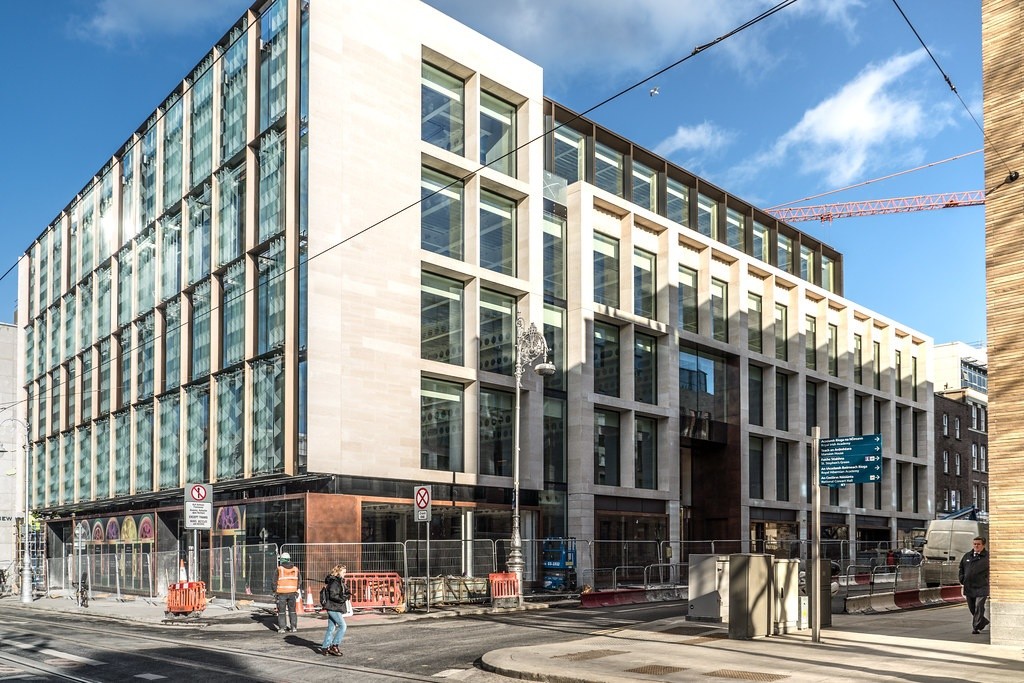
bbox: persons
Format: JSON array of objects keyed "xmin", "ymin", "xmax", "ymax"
[
  {"xmin": 886, "ymin": 550, "xmax": 899, "ymax": 573},
  {"xmin": 958, "ymin": 536, "xmax": 990, "ymax": 634},
  {"xmin": 321, "ymin": 564, "xmax": 351, "ymax": 656},
  {"xmin": 272, "ymin": 552, "xmax": 302, "ymax": 633}
]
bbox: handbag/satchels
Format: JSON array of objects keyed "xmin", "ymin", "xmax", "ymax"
[{"xmin": 341, "ymin": 599, "xmax": 353, "ymax": 617}]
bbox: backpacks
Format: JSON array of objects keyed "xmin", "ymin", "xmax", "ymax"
[{"xmin": 320, "ymin": 580, "xmax": 339, "ymax": 605}]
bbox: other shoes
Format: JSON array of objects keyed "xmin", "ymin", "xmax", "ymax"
[
  {"xmin": 979, "ymin": 620, "xmax": 989, "ymax": 630},
  {"xmin": 322, "ymin": 648, "xmax": 331, "ymax": 656},
  {"xmin": 278, "ymin": 629, "xmax": 285, "ymax": 634},
  {"xmin": 289, "ymin": 628, "xmax": 297, "ymax": 632},
  {"xmin": 972, "ymin": 629, "xmax": 979, "ymax": 634},
  {"xmin": 329, "ymin": 645, "xmax": 342, "ymax": 656}
]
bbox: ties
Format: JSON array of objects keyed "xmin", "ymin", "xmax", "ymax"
[{"xmin": 974, "ymin": 553, "xmax": 978, "ymax": 558}]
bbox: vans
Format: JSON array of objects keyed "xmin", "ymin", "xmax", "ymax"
[{"xmin": 922, "ymin": 519, "xmax": 991, "ymax": 588}]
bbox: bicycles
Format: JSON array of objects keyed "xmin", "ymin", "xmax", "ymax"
[{"xmin": 77, "ymin": 581, "xmax": 89, "ymax": 607}]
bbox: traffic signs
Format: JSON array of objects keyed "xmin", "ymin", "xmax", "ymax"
[{"xmin": 819, "ymin": 433, "xmax": 882, "ymax": 484}]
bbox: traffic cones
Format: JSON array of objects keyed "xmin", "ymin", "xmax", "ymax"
[
  {"xmin": 296, "ymin": 588, "xmax": 305, "ymax": 614},
  {"xmin": 180, "ymin": 558, "xmax": 187, "ymax": 583},
  {"xmin": 306, "ymin": 585, "xmax": 315, "ymax": 611}
]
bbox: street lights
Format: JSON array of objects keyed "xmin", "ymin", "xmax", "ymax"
[{"xmin": 506, "ymin": 316, "xmax": 556, "ymax": 604}]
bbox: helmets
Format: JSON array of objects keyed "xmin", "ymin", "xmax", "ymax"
[{"xmin": 281, "ymin": 552, "xmax": 290, "ymax": 559}]
